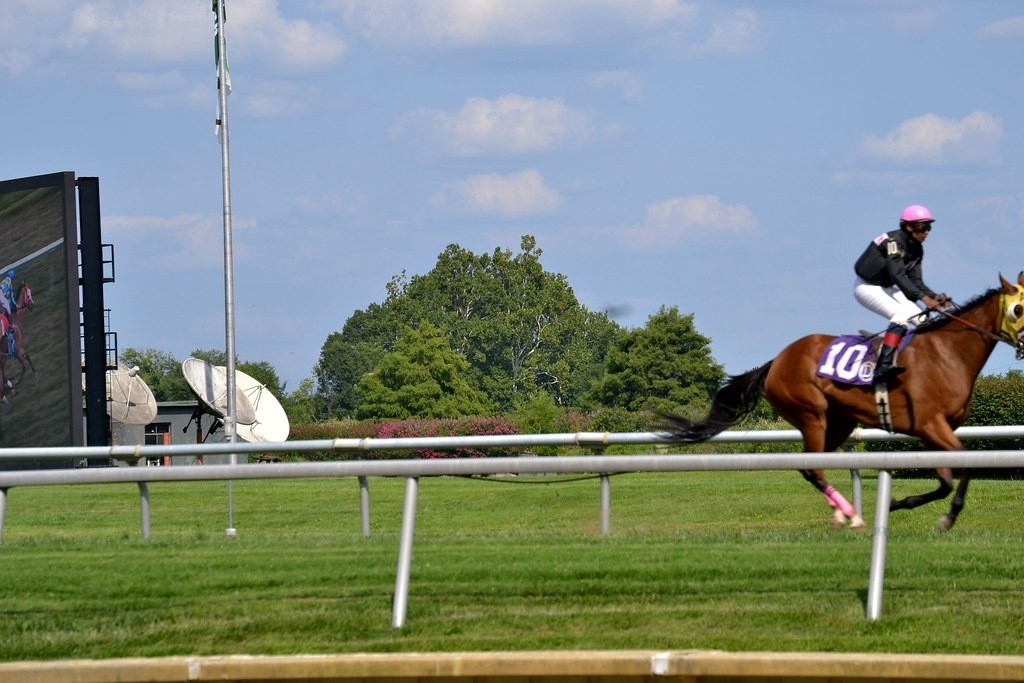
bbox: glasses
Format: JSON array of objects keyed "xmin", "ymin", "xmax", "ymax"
[{"xmin": 913, "ymin": 226, "xmax": 931, "ymax": 234}]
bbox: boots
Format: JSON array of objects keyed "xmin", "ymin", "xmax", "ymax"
[
  {"xmin": 8, "ymin": 314, "xmax": 16, "ymax": 333},
  {"xmin": 874, "ymin": 322, "xmax": 907, "ymax": 382}
]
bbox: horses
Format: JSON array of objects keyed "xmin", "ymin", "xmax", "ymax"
[
  {"xmin": 0, "ymin": 278, "xmax": 37, "ymax": 405},
  {"xmin": 648, "ymin": 270, "xmax": 1024, "ymax": 533}
]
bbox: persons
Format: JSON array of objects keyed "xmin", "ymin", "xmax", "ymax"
[
  {"xmin": 852, "ymin": 206, "xmax": 954, "ymax": 380},
  {"xmin": 0, "ymin": 270, "xmax": 18, "ymax": 335}
]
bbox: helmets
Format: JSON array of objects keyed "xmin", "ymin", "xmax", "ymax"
[
  {"xmin": 8, "ymin": 270, "xmax": 15, "ymax": 282},
  {"xmin": 900, "ymin": 205, "xmax": 935, "ymax": 225}
]
